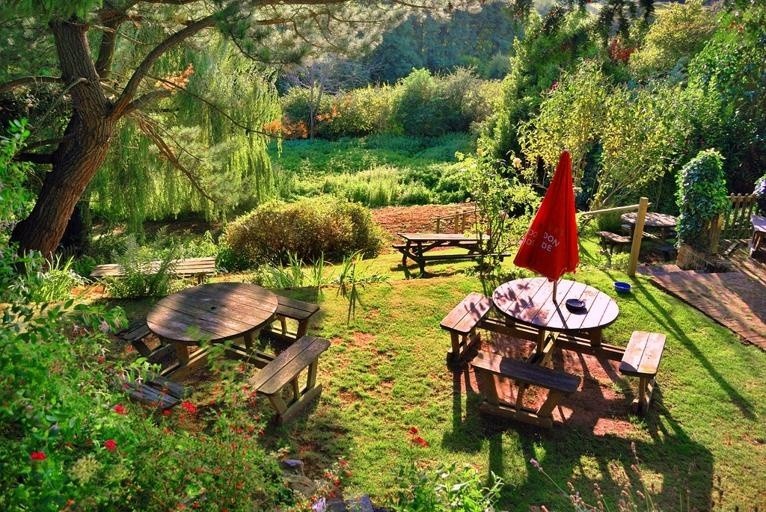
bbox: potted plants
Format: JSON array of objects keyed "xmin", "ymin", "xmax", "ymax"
[{"xmin": 671, "ymin": 146, "xmax": 732, "ymax": 273}]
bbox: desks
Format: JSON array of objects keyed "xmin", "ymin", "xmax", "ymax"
[
  {"xmin": 494, "ymin": 276, "xmax": 620, "ymax": 357},
  {"xmin": 146, "ymin": 282, "xmax": 278, "ymax": 361},
  {"xmin": 749, "ymin": 212, "xmax": 766, "ymax": 254}
]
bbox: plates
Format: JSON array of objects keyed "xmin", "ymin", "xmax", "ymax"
[{"xmin": 567, "ymin": 298, "xmax": 585, "ymax": 308}]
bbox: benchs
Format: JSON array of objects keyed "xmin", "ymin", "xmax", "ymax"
[
  {"xmin": 91, "ymin": 360, "xmax": 193, "ymax": 425},
  {"xmin": 440, "ymin": 288, "xmax": 497, "ymax": 355},
  {"xmin": 596, "ymin": 209, "xmax": 684, "ymax": 258},
  {"xmin": 392, "ymin": 228, "xmax": 512, "ymax": 272},
  {"xmin": 259, "ymin": 289, "xmax": 322, "ymax": 351},
  {"xmin": 123, "ymin": 316, "xmax": 186, "ymax": 360},
  {"xmin": 472, "ymin": 350, "xmax": 581, "ymax": 431},
  {"xmin": 89, "ymin": 255, "xmax": 217, "ymax": 289},
  {"xmin": 251, "ymin": 335, "xmax": 332, "ymax": 425},
  {"xmin": 618, "ymin": 327, "xmax": 673, "ymax": 415}
]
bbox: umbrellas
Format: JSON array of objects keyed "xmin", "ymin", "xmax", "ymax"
[{"xmin": 513, "ymin": 149, "xmax": 578, "ymax": 303}]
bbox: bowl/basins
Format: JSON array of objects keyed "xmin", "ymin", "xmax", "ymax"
[{"xmin": 612, "ymin": 282, "xmax": 631, "ymax": 293}]
